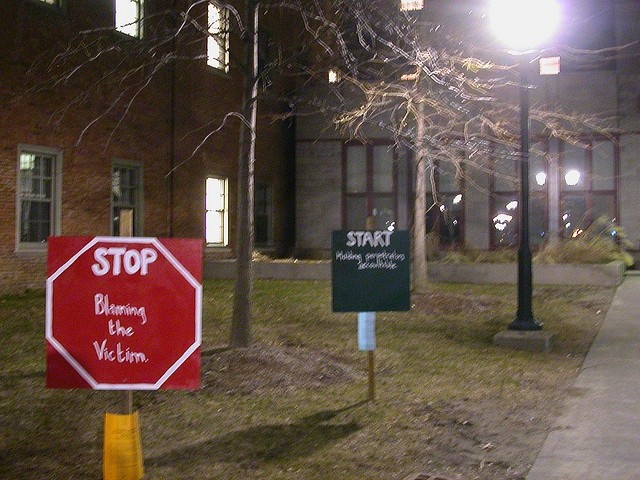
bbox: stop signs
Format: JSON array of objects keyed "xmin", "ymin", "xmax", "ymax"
[{"xmin": 46, "ymin": 236, "xmax": 204, "ymax": 390}]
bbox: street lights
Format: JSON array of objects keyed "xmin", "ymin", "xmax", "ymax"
[{"xmin": 490, "ymin": 0, "xmax": 561, "ymax": 330}]
